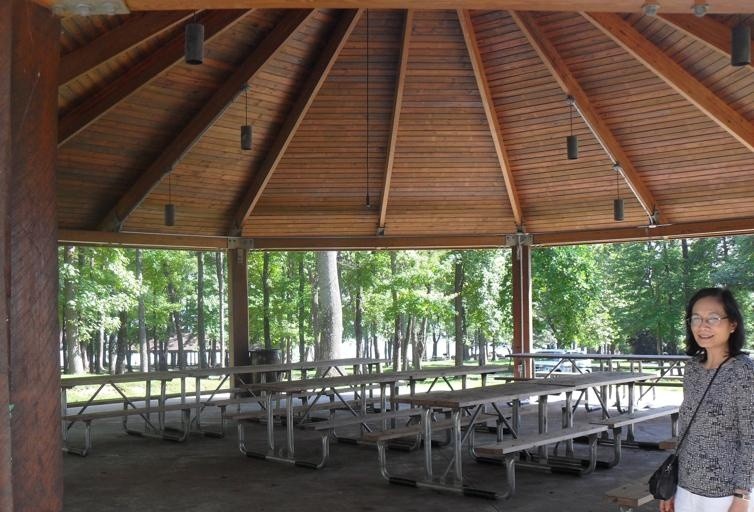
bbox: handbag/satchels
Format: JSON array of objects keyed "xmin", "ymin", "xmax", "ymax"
[{"xmin": 648, "ymin": 451, "xmax": 679, "ymax": 500}]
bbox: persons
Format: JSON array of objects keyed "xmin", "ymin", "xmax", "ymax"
[{"xmin": 657, "ymin": 284, "xmax": 754, "ymax": 512}]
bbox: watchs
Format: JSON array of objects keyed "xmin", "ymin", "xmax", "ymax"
[{"xmin": 733, "ymin": 492, "xmax": 751, "ymax": 502}]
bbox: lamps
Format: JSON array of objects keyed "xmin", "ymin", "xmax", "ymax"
[
  {"xmin": 563, "ymin": 95, "xmax": 581, "ymax": 161},
  {"xmin": 730, "ymin": 13, "xmax": 752, "ymax": 67},
  {"xmin": 239, "ymin": 86, "xmax": 253, "ymax": 152},
  {"xmin": 163, "ymin": 167, "xmax": 176, "ymax": 228},
  {"xmin": 183, "ymin": 10, "xmax": 204, "ymax": 65},
  {"xmin": 611, "ymin": 162, "xmax": 626, "ymax": 224}
]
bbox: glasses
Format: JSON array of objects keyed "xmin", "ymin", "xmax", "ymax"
[{"xmin": 686, "ymin": 314, "xmax": 729, "ymax": 328}]
